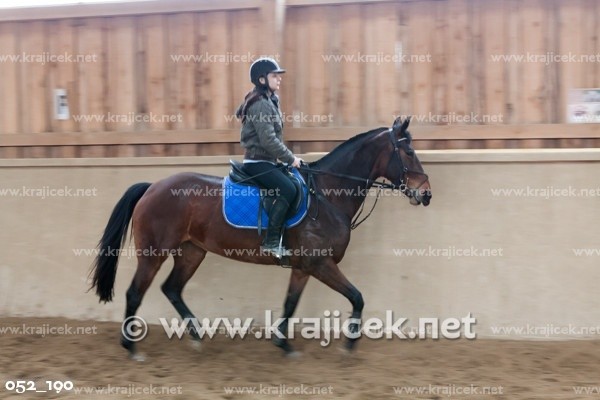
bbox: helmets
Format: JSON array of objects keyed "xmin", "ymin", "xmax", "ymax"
[{"xmin": 251, "ymin": 59, "xmax": 285, "ymax": 84}]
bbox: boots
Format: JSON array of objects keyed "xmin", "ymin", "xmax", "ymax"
[{"xmin": 261, "ymin": 195, "xmax": 291, "ymax": 252}]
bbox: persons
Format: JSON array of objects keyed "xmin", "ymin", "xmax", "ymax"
[{"xmin": 235, "ymin": 58, "xmax": 300, "ymax": 257}]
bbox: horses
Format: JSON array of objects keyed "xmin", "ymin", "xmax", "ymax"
[{"xmin": 85, "ymin": 115, "xmax": 433, "ymax": 362}]
